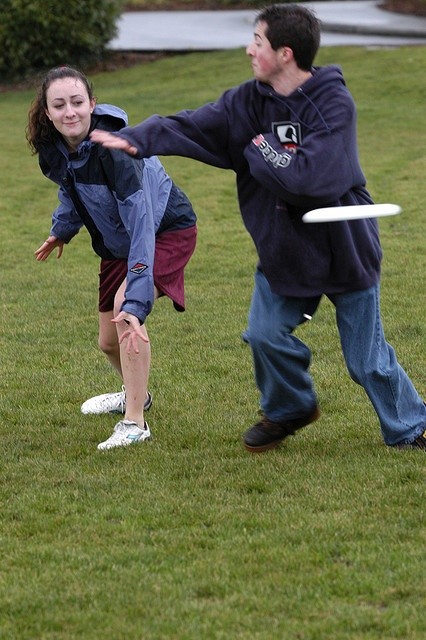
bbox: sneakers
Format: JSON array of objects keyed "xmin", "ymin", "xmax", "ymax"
[
  {"xmin": 97, "ymin": 419, "xmax": 151, "ymax": 451},
  {"xmin": 398, "ymin": 435, "xmax": 425, "ymax": 451},
  {"xmin": 245, "ymin": 401, "xmax": 320, "ymax": 452},
  {"xmin": 81, "ymin": 391, "xmax": 152, "ymax": 414}
]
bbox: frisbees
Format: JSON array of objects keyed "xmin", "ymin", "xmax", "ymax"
[{"xmin": 302, "ymin": 204, "xmax": 402, "ymax": 223}]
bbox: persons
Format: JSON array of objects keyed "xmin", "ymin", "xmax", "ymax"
[
  {"xmin": 88, "ymin": 4, "xmax": 425, "ymax": 457},
  {"xmin": 22, "ymin": 64, "xmax": 199, "ymax": 455}
]
